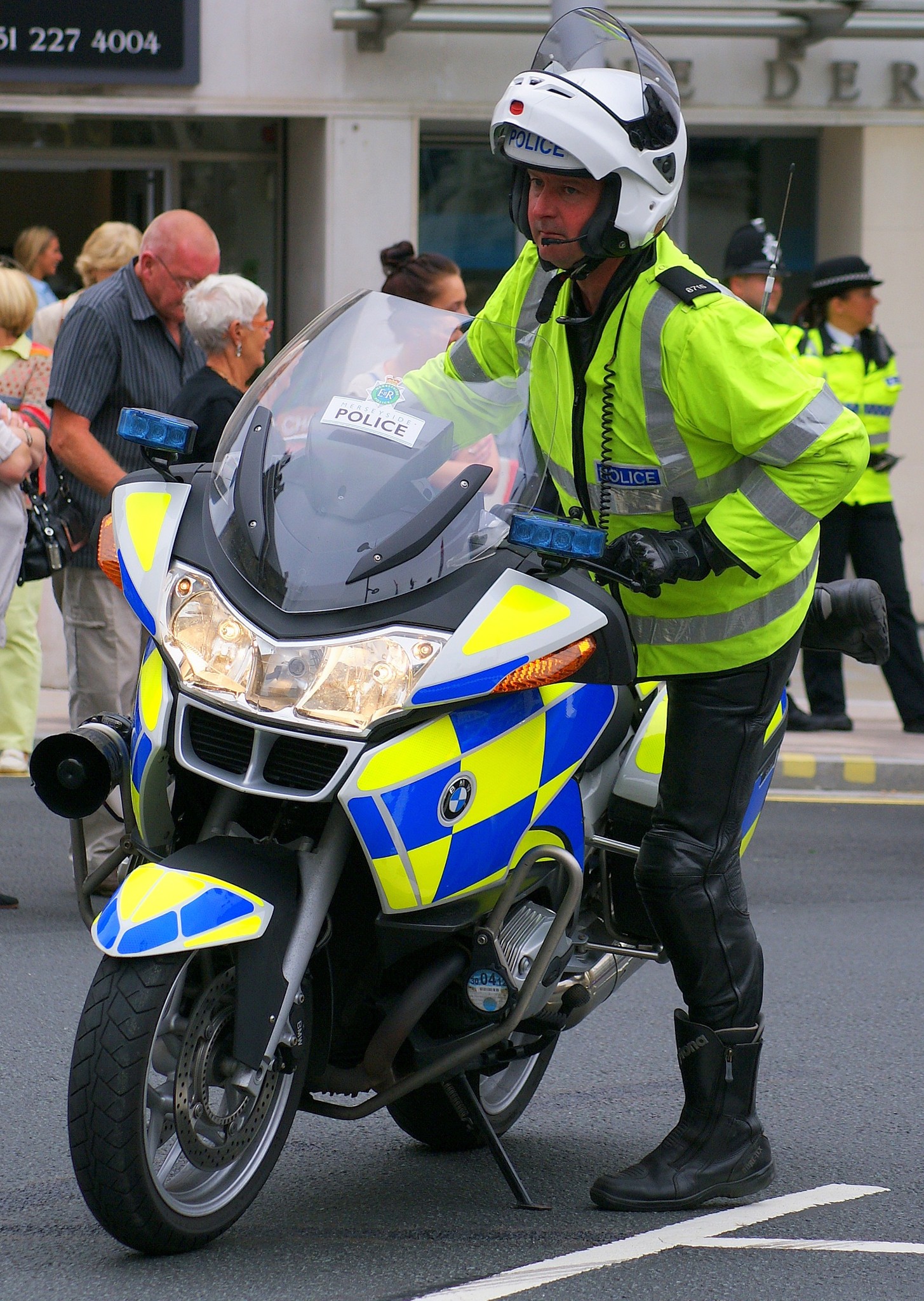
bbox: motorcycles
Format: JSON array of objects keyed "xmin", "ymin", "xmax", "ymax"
[{"xmin": 14, "ymin": 284, "xmax": 799, "ymax": 1261}]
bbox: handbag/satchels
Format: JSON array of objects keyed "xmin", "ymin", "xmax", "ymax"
[{"xmin": 18, "ymin": 441, "xmax": 88, "ymax": 586}]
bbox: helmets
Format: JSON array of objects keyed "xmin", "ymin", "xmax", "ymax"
[
  {"xmin": 720, "ymin": 218, "xmax": 789, "ymax": 281},
  {"xmin": 486, "ymin": 68, "xmax": 685, "ymax": 254}
]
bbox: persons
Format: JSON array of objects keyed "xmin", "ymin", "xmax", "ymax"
[
  {"xmin": 348, "ymin": 241, "xmax": 501, "ymax": 490},
  {"xmin": 46, "ymin": 207, "xmax": 222, "ymax": 895},
  {"xmin": 360, "ymin": 66, "xmax": 870, "ymax": 1210},
  {"xmin": 155, "ymin": 274, "xmax": 285, "ymax": 462},
  {"xmin": 706, "ymin": 218, "xmax": 924, "ymax": 738},
  {"xmin": 0, "ymin": 220, "xmax": 146, "ymax": 779}
]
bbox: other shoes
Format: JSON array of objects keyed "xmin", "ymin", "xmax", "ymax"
[
  {"xmin": 807, "ymin": 709, "xmax": 853, "ymax": 733},
  {"xmin": 96, "ymin": 871, "xmax": 120, "ymax": 898},
  {"xmin": 0, "ymin": 747, "xmax": 31, "ymax": 781},
  {"xmin": 0, "ymin": 891, "xmax": 19, "ymax": 909},
  {"xmin": 784, "ymin": 698, "xmax": 818, "ymax": 728}
]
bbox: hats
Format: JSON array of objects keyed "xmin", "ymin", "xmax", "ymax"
[{"xmin": 806, "ymin": 253, "xmax": 885, "ymax": 291}]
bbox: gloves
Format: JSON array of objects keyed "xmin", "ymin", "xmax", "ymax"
[{"xmin": 591, "ymin": 496, "xmax": 710, "ymax": 594}]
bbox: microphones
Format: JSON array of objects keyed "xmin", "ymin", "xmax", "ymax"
[{"xmin": 541, "ymin": 234, "xmax": 587, "ymax": 246}]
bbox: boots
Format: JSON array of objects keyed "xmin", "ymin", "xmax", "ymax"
[
  {"xmin": 805, "ymin": 577, "xmax": 890, "ymax": 666},
  {"xmin": 587, "ymin": 1008, "xmax": 774, "ymax": 1211}
]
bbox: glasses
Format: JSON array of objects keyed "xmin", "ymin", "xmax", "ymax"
[
  {"xmin": 151, "ymin": 254, "xmax": 199, "ymax": 290},
  {"xmin": 220, "ymin": 321, "xmax": 276, "ymax": 341}
]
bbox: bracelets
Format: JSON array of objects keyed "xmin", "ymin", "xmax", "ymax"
[
  {"xmin": 6, "ymin": 406, "xmax": 11, "ymax": 425},
  {"xmin": 22, "ymin": 427, "xmax": 32, "ymax": 447}
]
